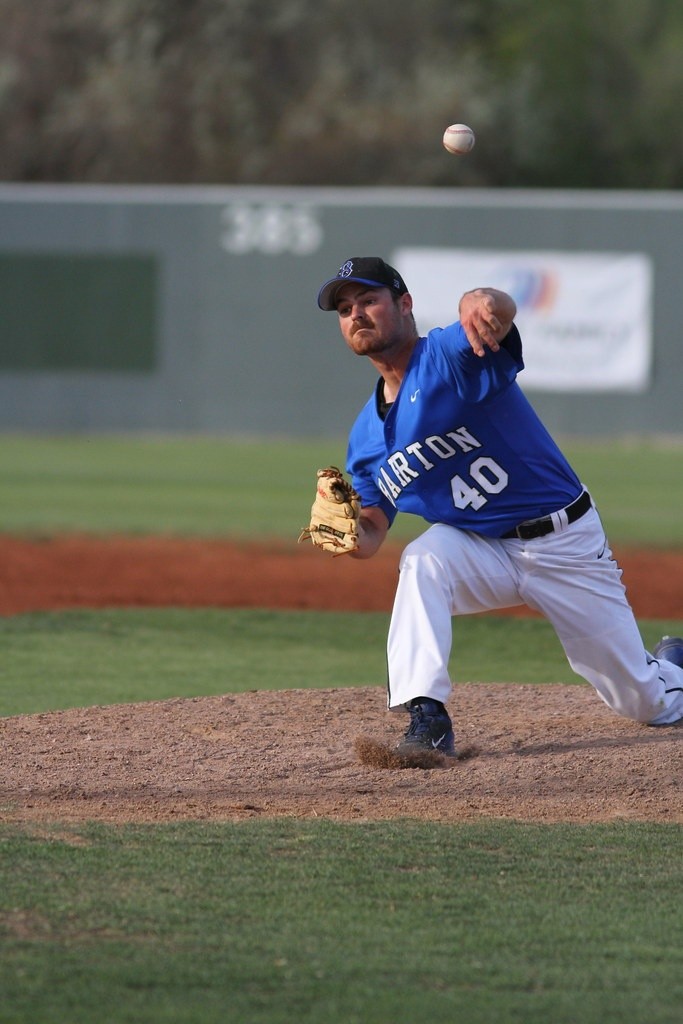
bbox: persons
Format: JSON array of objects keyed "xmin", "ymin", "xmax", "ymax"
[{"xmin": 296, "ymin": 257, "xmax": 683, "ymax": 769}]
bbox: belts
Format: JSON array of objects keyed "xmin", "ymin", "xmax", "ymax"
[{"xmin": 501, "ymin": 492, "xmax": 591, "ymax": 541}]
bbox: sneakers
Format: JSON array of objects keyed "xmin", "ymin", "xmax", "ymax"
[
  {"xmin": 393, "ymin": 707, "xmax": 455, "ymax": 764},
  {"xmin": 654, "ymin": 635, "xmax": 683, "ymax": 667}
]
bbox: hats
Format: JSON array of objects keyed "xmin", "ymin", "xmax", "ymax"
[{"xmin": 318, "ymin": 259, "xmax": 408, "ymax": 311}]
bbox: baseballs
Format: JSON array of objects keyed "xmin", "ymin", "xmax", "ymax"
[{"xmin": 442, "ymin": 123, "xmax": 476, "ymax": 157}]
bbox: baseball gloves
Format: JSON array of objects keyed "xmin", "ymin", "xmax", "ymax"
[{"xmin": 309, "ymin": 468, "xmax": 361, "ymax": 554}]
